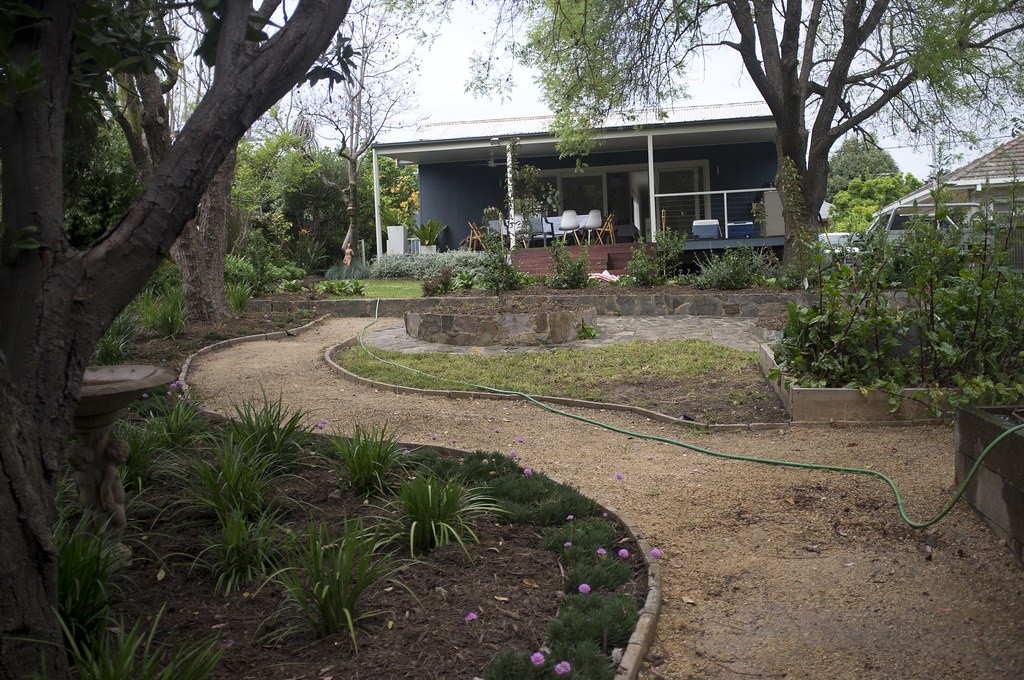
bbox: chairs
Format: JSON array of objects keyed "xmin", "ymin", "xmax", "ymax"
[
  {"xmin": 468, "ymin": 221, "xmax": 487, "ymax": 251},
  {"xmin": 594, "ymin": 214, "xmax": 616, "ymax": 244},
  {"xmin": 559, "ymin": 210, "xmax": 581, "ymax": 246},
  {"xmin": 527, "ymin": 209, "xmax": 556, "ymax": 247},
  {"xmin": 581, "ymin": 209, "xmax": 602, "ymax": 245}
]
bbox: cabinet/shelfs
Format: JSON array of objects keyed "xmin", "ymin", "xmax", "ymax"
[{"xmin": 759, "ymin": 190, "xmax": 786, "ymax": 237}]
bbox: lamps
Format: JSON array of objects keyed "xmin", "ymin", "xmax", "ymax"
[{"xmin": 490, "ymin": 137, "xmax": 499, "ymax": 145}]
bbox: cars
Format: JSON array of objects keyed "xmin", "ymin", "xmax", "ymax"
[{"xmin": 819, "ymin": 233, "xmax": 871, "ymax": 273}]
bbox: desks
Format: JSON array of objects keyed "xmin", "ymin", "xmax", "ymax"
[{"xmin": 489, "ymin": 215, "xmax": 589, "ymax": 243}]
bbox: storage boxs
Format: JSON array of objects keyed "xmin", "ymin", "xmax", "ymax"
[
  {"xmin": 691, "ymin": 219, "xmax": 720, "ymax": 240},
  {"xmin": 728, "ymin": 221, "xmax": 755, "ymax": 239}
]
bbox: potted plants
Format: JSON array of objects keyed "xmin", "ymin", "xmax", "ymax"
[
  {"xmin": 377, "ymin": 204, "xmax": 411, "ymax": 255},
  {"xmin": 408, "ymin": 219, "xmax": 448, "ymax": 255}
]
